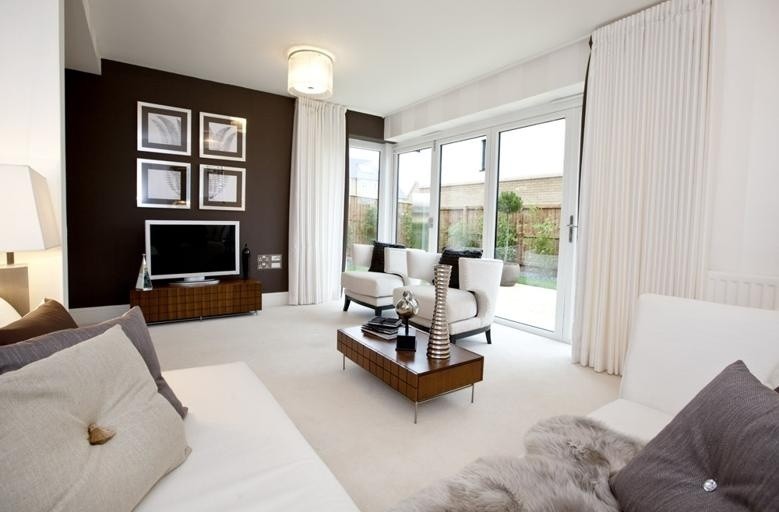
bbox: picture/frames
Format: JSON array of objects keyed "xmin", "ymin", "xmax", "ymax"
[
  {"xmin": 198, "ymin": 164, "xmax": 246, "ymax": 212},
  {"xmin": 137, "ymin": 158, "xmax": 191, "ymax": 209},
  {"xmin": 137, "ymin": 101, "xmax": 191, "ymax": 156},
  {"xmin": 199, "ymin": 112, "xmax": 247, "ymax": 162}
]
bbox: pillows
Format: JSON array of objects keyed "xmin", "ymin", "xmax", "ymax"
[
  {"xmin": 368, "ymin": 241, "xmax": 405, "ymax": 272},
  {"xmin": 0, "ymin": 323, "xmax": 192, "ymax": 512},
  {"xmin": 432, "ymin": 249, "xmax": 483, "ymax": 289},
  {"xmin": 608, "ymin": 359, "xmax": 779, "ymax": 512},
  {"xmin": 0, "ymin": 305, "xmax": 189, "ymax": 420},
  {"xmin": 0, "ymin": 298, "xmax": 79, "ymax": 346}
]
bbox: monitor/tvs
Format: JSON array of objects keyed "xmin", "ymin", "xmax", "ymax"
[{"xmin": 145, "ymin": 220, "xmax": 240, "ymax": 286}]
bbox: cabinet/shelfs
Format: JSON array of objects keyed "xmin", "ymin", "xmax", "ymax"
[{"xmin": 130, "ymin": 277, "xmax": 263, "ymax": 326}]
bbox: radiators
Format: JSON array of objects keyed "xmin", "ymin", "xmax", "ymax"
[{"xmin": 701, "ymin": 272, "xmax": 779, "ymax": 311}]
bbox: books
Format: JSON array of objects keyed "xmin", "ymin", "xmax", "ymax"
[{"xmin": 361, "ymin": 316, "xmax": 404, "ymax": 341}]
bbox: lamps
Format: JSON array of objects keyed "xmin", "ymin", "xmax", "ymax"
[
  {"xmin": 287, "ymin": 45, "xmax": 335, "ymax": 100},
  {"xmin": 1, "ymin": 163, "xmax": 62, "ymax": 318}
]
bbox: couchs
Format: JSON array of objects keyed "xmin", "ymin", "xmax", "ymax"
[
  {"xmin": 0, "ymin": 294, "xmax": 361, "ymax": 512},
  {"xmin": 391, "ymin": 294, "xmax": 779, "ymax": 512},
  {"xmin": 341, "ymin": 244, "xmax": 425, "ymax": 316},
  {"xmin": 392, "ymin": 249, "xmax": 504, "ymax": 345}
]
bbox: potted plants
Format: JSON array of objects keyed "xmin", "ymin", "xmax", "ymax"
[{"xmin": 497, "ymin": 190, "xmax": 523, "ymax": 287}]
